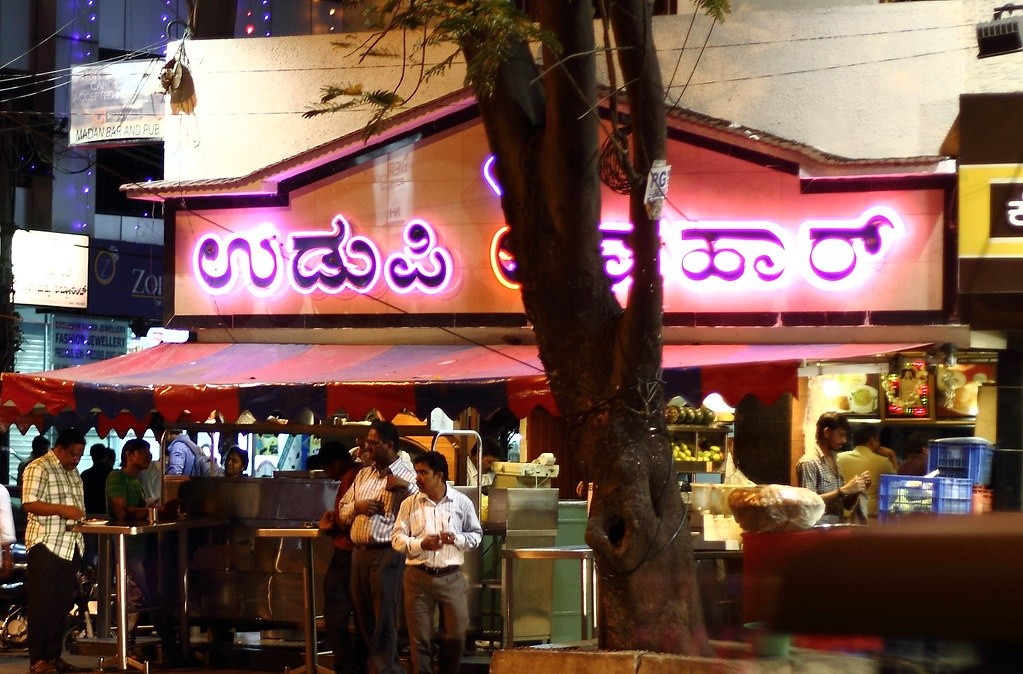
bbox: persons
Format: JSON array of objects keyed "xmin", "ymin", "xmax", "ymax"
[
  {"xmin": 795, "ymin": 411, "xmax": 930, "ymax": 526},
  {"xmin": 0, "ymin": 417, "xmax": 500, "ymax": 674}
]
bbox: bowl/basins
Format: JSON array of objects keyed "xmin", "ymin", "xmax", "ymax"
[
  {"xmin": 744, "ymin": 623, "xmax": 791, "ymax": 655},
  {"xmin": 127, "ymin": 507, "xmax": 149, "ymax": 521}
]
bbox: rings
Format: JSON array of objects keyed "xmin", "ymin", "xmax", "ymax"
[{"xmin": 448, "ymin": 537, "xmax": 451, "ymax": 540}]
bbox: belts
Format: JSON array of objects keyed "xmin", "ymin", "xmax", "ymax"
[
  {"xmin": 354, "ymin": 543, "xmax": 386, "ymax": 550},
  {"xmin": 417, "ymin": 563, "xmax": 459, "ymax": 576}
]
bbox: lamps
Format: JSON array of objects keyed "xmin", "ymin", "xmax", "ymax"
[{"xmin": 976, "ymin": 5, "xmax": 1023, "ymax": 59}]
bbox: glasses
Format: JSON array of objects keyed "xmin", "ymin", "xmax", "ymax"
[{"xmin": 365, "ymin": 439, "xmax": 387, "ymax": 445}]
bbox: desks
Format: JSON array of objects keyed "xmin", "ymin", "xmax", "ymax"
[
  {"xmin": 255, "ymin": 527, "xmax": 337, "ymax": 674},
  {"xmin": 500, "ymin": 543, "xmax": 745, "ymax": 641},
  {"xmin": 64, "ymin": 516, "xmax": 232, "ymax": 674}
]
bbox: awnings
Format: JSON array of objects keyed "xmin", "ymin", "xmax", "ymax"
[{"xmin": 0, "ymin": 340, "xmax": 930, "ymax": 505}]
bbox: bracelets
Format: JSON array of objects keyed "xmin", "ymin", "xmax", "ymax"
[{"xmin": 838, "ymin": 488, "xmax": 848, "ymax": 500}]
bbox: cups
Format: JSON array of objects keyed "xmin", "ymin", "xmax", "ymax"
[{"xmin": 147, "ymin": 507, "xmax": 158, "ymax": 525}]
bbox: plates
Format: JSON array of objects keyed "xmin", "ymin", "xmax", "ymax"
[{"xmin": 82, "ymin": 521, "xmax": 109, "ymax": 525}]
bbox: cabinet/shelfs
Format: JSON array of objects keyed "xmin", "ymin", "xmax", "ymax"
[{"xmin": 668, "ymin": 423, "xmax": 734, "ymax": 484}]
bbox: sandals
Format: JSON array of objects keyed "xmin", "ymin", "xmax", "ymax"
[{"xmin": 29, "ymin": 658, "xmax": 78, "ymax": 674}]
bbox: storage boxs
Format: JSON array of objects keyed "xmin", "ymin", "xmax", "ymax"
[
  {"xmin": 927, "ymin": 441, "xmax": 996, "ymax": 488},
  {"xmin": 971, "ymin": 484, "xmax": 994, "ymax": 515},
  {"xmin": 878, "ymin": 474, "xmax": 973, "ymax": 516}
]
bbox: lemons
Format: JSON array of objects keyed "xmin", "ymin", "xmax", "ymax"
[{"xmin": 670, "ymin": 439, "xmax": 723, "ymax": 462}]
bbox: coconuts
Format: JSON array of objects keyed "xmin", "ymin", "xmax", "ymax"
[{"xmin": 665, "ymin": 405, "xmax": 717, "ymax": 425}]
